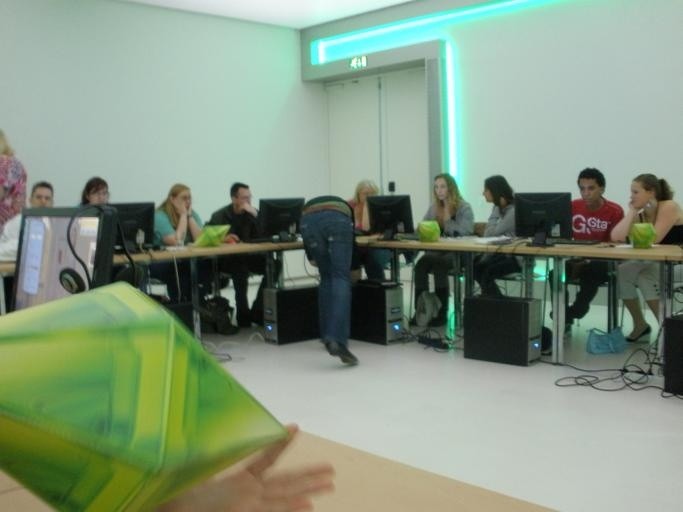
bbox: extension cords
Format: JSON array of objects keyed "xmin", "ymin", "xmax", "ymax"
[{"xmin": 418, "ymin": 336, "xmax": 452, "ymax": 349}]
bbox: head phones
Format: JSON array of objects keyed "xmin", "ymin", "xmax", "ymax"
[{"xmin": 59, "ymin": 203, "xmax": 145, "ymax": 294}]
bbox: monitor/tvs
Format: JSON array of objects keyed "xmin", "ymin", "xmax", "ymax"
[
  {"xmin": 9, "ymin": 206, "xmax": 117, "ymax": 313},
  {"xmin": 259, "ymin": 198, "xmax": 306, "ymax": 242},
  {"xmin": 514, "ymin": 193, "xmax": 571, "ymax": 246},
  {"xmin": 366, "ymin": 195, "xmax": 414, "ymax": 240},
  {"xmin": 92, "ymin": 202, "xmax": 155, "ymax": 246}
]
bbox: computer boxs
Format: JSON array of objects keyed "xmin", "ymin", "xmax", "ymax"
[
  {"xmin": 665, "ymin": 314, "xmax": 683, "ymax": 396},
  {"xmin": 350, "ymin": 281, "xmax": 404, "ymax": 345},
  {"xmin": 263, "ymin": 284, "xmax": 320, "ymax": 345},
  {"xmin": 464, "ymin": 294, "xmax": 542, "ymax": 367}
]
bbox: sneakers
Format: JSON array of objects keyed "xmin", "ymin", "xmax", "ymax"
[
  {"xmin": 409, "ymin": 308, "xmax": 450, "ymax": 328},
  {"xmin": 234, "ymin": 305, "xmax": 263, "ymax": 328},
  {"xmin": 549, "ymin": 310, "xmax": 575, "ymax": 342}
]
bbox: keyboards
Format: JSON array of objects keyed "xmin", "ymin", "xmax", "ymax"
[
  {"xmin": 243, "ymin": 238, "xmax": 279, "ymax": 243},
  {"xmin": 552, "ymin": 239, "xmax": 600, "ymax": 244},
  {"xmin": 399, "ymin": 232, "xmax": 420, "ymax": 239}
]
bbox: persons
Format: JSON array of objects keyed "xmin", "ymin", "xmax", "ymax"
[
  {"xmin": 548, "ymin": 167, "xmax": 682, "ymax": 342},
  {"xmin": 297, "ymin": 180, "xmax": 393, "ymax": 364},
  {"xmin": 152, "ymin": 424, "xmax": 336, "ymax": 511},
  {"xmin": 0, "ymin": 130, "xmax": 110, "ymax": 314},
  {"xmin": 150, "ymin": 183, "xmax": 282, "ymax": 335},
  {"xmin": 411, "ymin": 174, "xmax": 523, "ymax": 327}
]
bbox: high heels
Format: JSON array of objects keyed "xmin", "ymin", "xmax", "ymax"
[{"xmin": 624, "ymin": 324, "xmax": 652, "ymax": 343}]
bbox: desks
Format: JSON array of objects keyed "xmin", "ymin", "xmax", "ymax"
[{"xmin": 0, "ymin": 391, "xmax": 561, "ymax": 512}]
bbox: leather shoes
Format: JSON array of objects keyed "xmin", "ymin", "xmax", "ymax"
[{"xmin": 326, "ymin": 338, "xmax": 357, "ymax": 365}]
